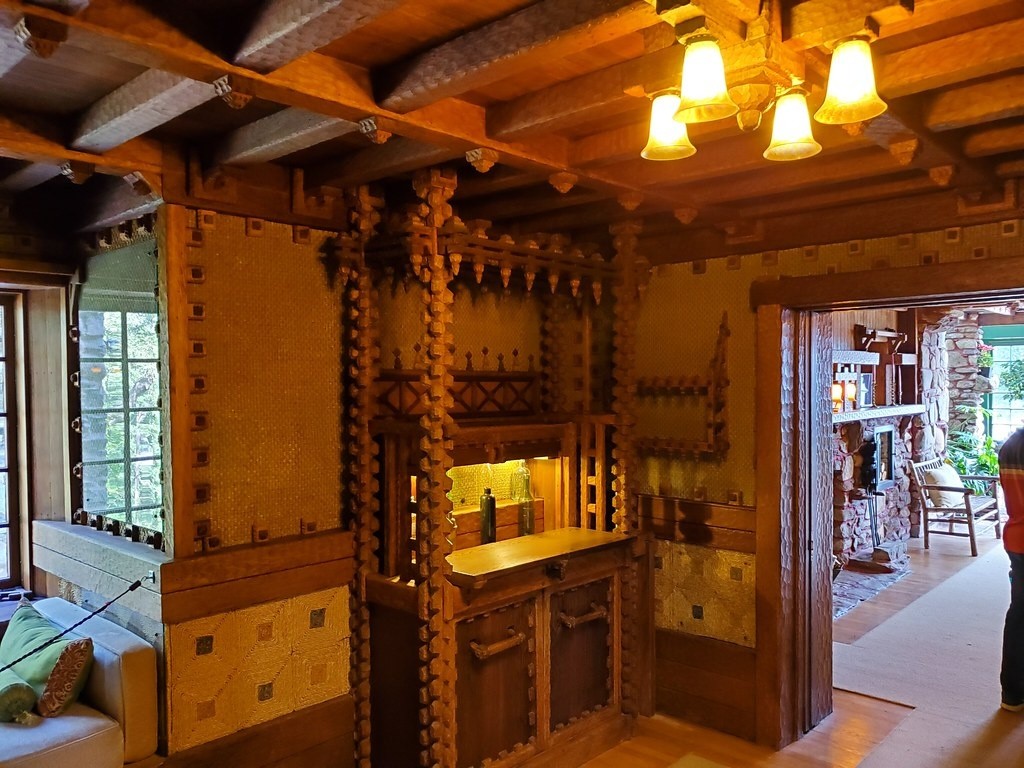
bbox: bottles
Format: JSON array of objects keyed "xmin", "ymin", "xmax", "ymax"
[
  {"xmin": 447, "ymin": 511, "xmax": 456, "ymax": 552},
  {"xmin": 517, "ymin": 474, "xmax": 536, "ymax": 538},
  {"xmin": 479, "ymin": 488, "xmax": 497, "ymax": 545}
]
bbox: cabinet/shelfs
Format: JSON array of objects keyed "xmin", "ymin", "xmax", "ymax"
[{"xmin": 375, "ymin": 549, "xmax": 622, "ymax": 768}]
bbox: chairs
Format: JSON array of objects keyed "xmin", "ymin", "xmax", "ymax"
[{"xmin": 910, "ymin": 452, "xmax": 1004, "ymax": 558}]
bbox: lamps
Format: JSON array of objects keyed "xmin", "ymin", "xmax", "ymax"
[
  {"xmin": 763, "ymin": 88, "xmax": 824, "ymax": 164},
  {"xmin": 815, "ymin": 37, "xmax": 891, "ymax": 129},
  {"xmin": 640, "ymin": 88, "xmax": 699, "ymax": 163},
  {"xmin": 670, "ymin": 32, "xmax": 741, "ymax": 126}
]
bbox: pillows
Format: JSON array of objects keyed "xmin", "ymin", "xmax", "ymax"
[
  {"xmin": 922, "ymin": 463, "xmax": 971, "ymax": 508},
  {"xmin": 0, "ymin": 597, "xmax": 95, "ymax": 718},
  {"xmin": 1, "ymin": 660, "xmax": 44, "ymax": 726}
]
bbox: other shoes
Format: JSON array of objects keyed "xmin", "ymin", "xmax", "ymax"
[{"xmin": 1000, "ymin": 694, "xmax": 1024, "ymax": 712}]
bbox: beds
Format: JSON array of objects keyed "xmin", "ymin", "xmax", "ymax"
[{"xmin": 0, "ymin": 595, "xmax": 158, "ymax": 768}]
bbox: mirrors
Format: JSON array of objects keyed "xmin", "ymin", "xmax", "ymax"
[{"xmin": 69, "ymin": 215, "xmax": 164, "ymax": 551}]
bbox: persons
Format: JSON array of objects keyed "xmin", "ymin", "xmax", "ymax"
[{"xmin": 998, "ymin": 427, "xmax": 1024, "ymax": 710}]
